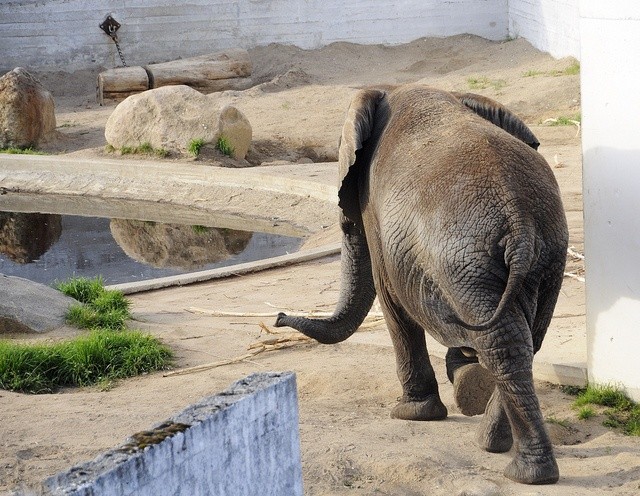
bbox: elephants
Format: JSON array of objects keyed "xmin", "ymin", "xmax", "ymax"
[{"xmin": 271, "ymin": 82, "xmax": 570, "ymax": 486}]
